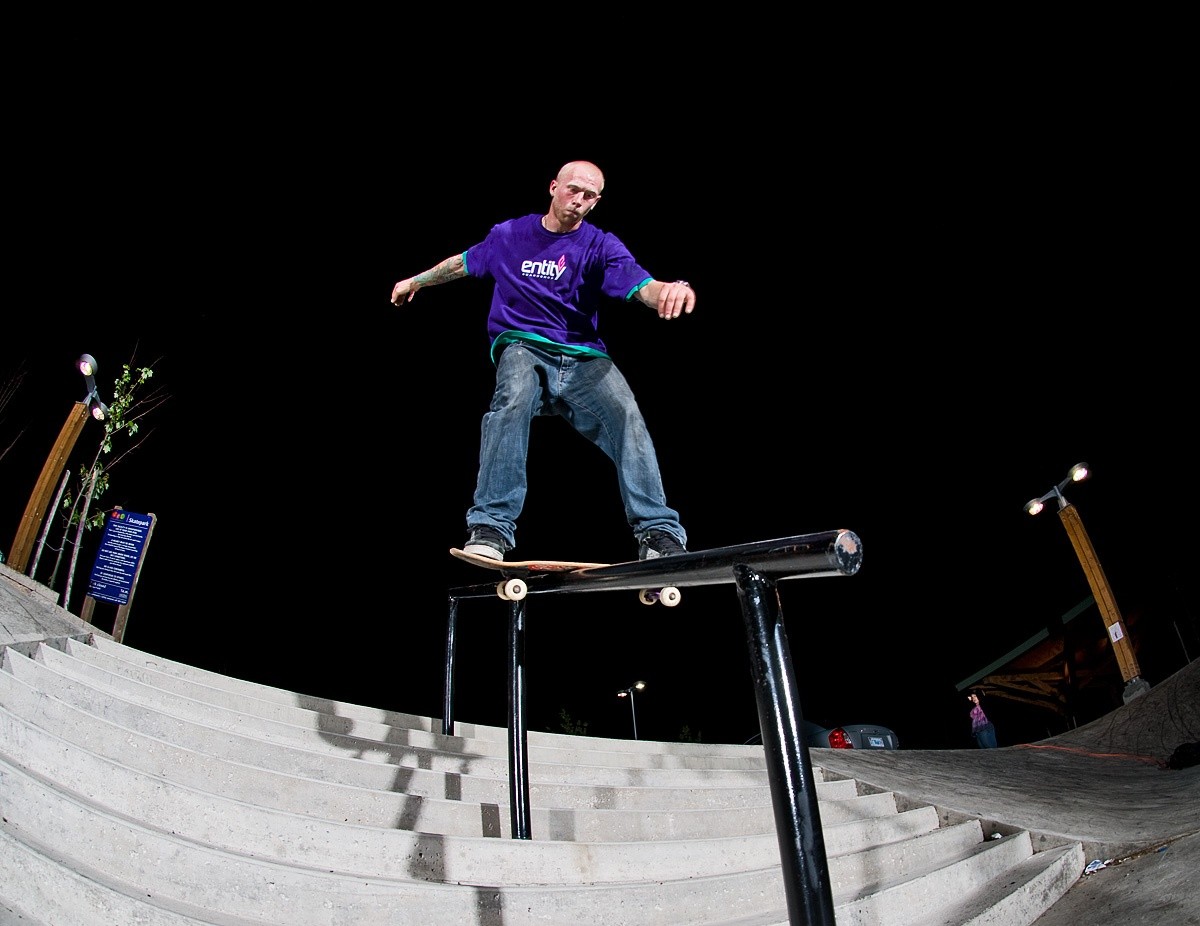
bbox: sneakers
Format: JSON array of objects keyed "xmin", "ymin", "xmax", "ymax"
[
  {"xmin": 636, "ymin": 529, "xmax": 689, "ymax": 561},
  {"xmin": 461, "ymin": 523, "xmax": 513, "ymax": 561}
]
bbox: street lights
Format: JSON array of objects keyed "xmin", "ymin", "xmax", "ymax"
[
  {"xmin": 9, "ymin": 351, "xmax": 110, "ymax": 575},
  {"xmin": 1022, "ymin": 461, "xmax": 1149, "ymax": 707},
  {"xmin": 616, "ymin": 679, "xmax": 647, "ymax": 741}
]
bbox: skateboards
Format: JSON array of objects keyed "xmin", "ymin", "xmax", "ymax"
[{"xmin": 449, "ymin": 547, "xmax": 681, "ymax": 607}]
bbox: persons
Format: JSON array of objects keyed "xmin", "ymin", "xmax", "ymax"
[
  {"xmin": 391, "ymin": 161, "xmax": 696, "ymax": 561},
  {"xmin": 970, "ymin": 692, "xmax": 997, "ymax": 749}
]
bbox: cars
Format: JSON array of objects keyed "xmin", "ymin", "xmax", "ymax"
[{"xmin": 742, "ymin": 718, "xmax": 900, "ymax": 750}]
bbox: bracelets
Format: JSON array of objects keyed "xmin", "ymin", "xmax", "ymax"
[{"xmin": 677, "ymin": 280, "xmax": 688, "ymax": 286}]
